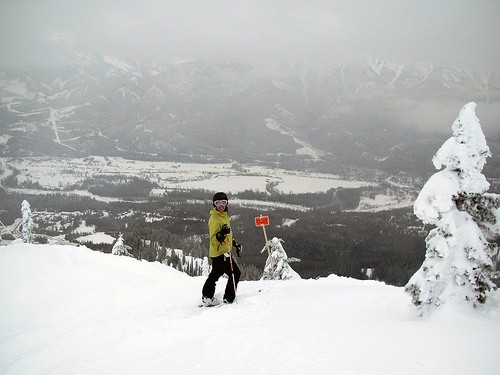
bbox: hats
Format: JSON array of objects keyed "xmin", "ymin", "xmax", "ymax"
[{"xmin": 213, "ymin": 192, "xmax": 228, "ymax": 207}]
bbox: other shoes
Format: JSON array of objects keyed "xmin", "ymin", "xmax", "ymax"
[{"xmin": 202, "ymin": 296, "xmax": 215, "ymax": 303}]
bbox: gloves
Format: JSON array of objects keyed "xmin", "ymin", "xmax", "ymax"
[
  {"xmin": 216, "ymin": 224, "xmax": 230, "ymax": 242},
  {"xmin": 233, "ymin": 240, "xmax": 241, "ymax": 252}
]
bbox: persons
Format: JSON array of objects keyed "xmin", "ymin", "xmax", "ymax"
[{"xmin": 201, "ymin": 192, "xmax": 241, "ymax": 305}]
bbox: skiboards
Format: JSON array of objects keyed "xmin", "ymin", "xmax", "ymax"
[{"xmin": 198, "ymin": 288, "xmax": 262, "ymax": 308}]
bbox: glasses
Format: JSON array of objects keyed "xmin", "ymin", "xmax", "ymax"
[{"xmin": 214, "ymin": 199, "xmax": 227, "ymax": 207}]
